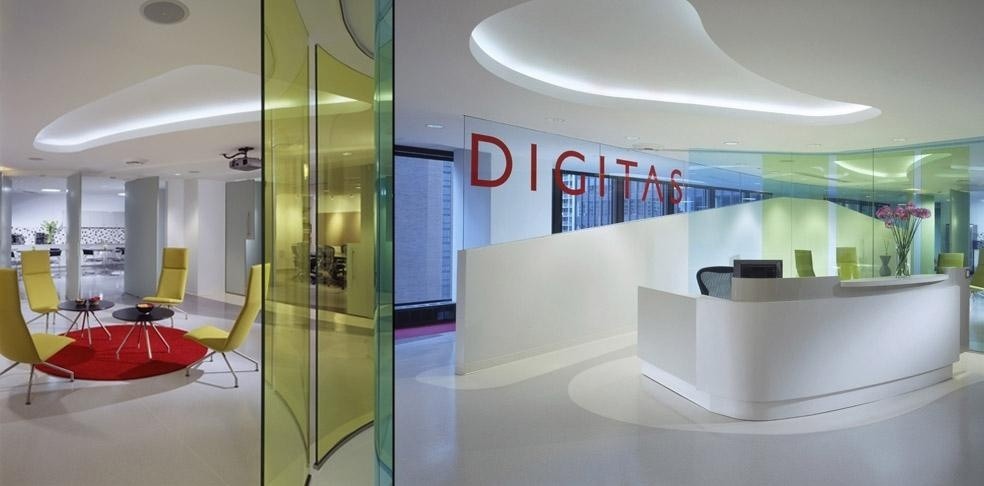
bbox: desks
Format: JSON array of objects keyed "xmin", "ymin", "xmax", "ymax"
[{"xmin": 55, "ymin": 299, "xmax": 174, "ymax": 361}]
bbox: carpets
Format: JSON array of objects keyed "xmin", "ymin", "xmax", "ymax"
[{"xmin": 36, "ymin": 326, "xmax": 208, "ymax": 381}]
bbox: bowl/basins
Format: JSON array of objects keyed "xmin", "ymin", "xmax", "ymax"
[
  {"xmin": 76, "ymin": 297, "xmax": 100, "ymax": 306},
  {"xmin": 136, "ymin": 303, "xmax": 153, "ymax": 315}
]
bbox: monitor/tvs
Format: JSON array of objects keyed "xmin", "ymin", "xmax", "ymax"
[{"xmin": 733, "ymin": 259, "xmax": 783, "ymax": 278}]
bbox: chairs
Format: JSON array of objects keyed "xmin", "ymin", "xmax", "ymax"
[
  {"xmin": 938, "ymin": 251, "xmax": 984, "ymax": 291},
  {"xmin": 291, "ymin": 242, "xmax": 347, "ymax": 291},
  {"xmin": 792, "ymin": 246, "xmax": 860, "ymax": 280},
  {"xmin": 140, "ymin": 246, "xmax": 271, "ymax": 390},
  {"xmin": 0, "ymin": 248, "xmax": 80, "ymax": 405}
]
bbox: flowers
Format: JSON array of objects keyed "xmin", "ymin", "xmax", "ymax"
[{"xmin": 875, "ymin": 200, "xmax": 931, "ymax": 277}]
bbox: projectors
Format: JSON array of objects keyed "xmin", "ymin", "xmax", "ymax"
[{"xmin": 228, "ymin": 158, "xmax": 262, "ymax": 171}]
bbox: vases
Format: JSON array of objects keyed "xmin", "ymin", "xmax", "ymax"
[
  {"xmin": 895, "ymin": 246, "xmax": 910, "ymax": 277},
  {"xmin": 879, "ymin": 254, "xmax": 892, "ymax": 276}
]
